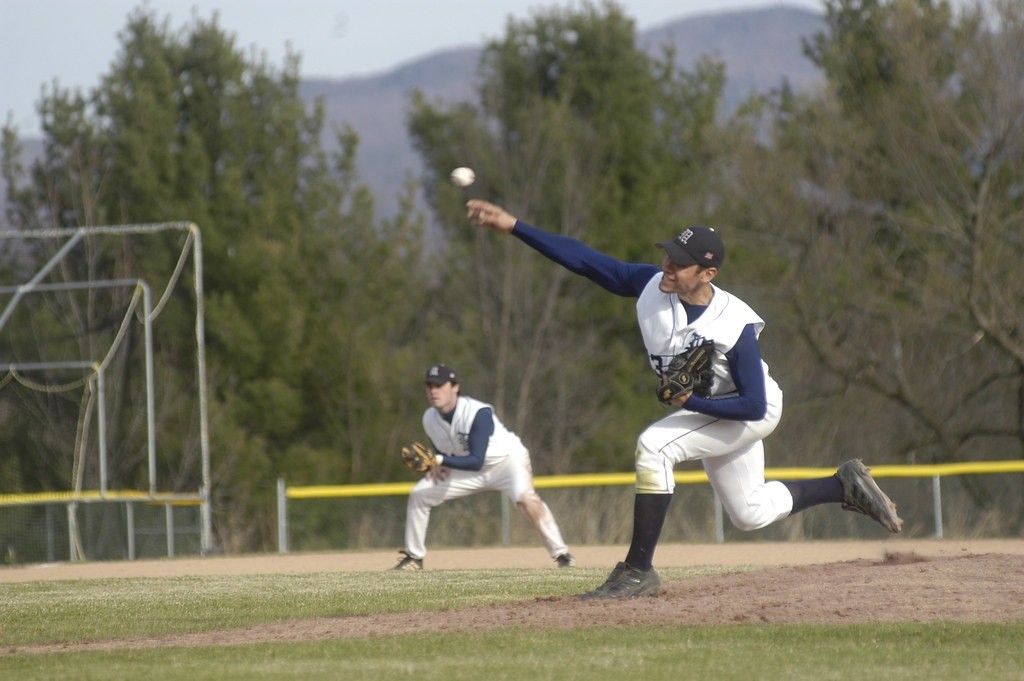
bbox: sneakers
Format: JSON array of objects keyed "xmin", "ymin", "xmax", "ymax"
[
  {"xmin": 834, "ymin": 458, "xmax": 903, "ymax": 534},
  {"xmin": 556, "ymin": 553, "xmax": 578, "ymax": 570},
  {"xmin": 390, "ymin": 549, "xmax": 424, "ymax": 572},
  {"xmin": 577, "ymin": 562, "xmax": 663, "ymax": 607}
]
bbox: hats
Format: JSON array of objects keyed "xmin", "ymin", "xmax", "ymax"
[
  {"xmin": 424, "ymin": 364, "xmax": 458, "ymax": 386},
  {"xmin": 655, "ymin": 227, "xmax": 727, "ymax": 270}
]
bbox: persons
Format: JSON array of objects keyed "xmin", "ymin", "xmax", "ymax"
[
  {"xmin": 389, "ymin": 364, "xmax": 575, "ymax": 571},
  {"xmin": 467, "ymin": 198, "xmax": 903, "ymax": 596}
]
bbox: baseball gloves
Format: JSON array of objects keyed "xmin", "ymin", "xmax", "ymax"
[
  {"xmin": 657, "ymin": 343, "xmax": 715, "ymax": 404},
  {"xmin": 401, "ymin": 442, "xmax": 436, "ymax": 474}
]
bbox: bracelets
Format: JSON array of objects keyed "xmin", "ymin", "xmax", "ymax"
[{"xmin": 435, "ymin": 455, "xmax": 443, "ymax": 466}]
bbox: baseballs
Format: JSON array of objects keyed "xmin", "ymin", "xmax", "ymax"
[{"xmin": 450, "ymin": 167, "xmax": 475, "ymax": 187}]
